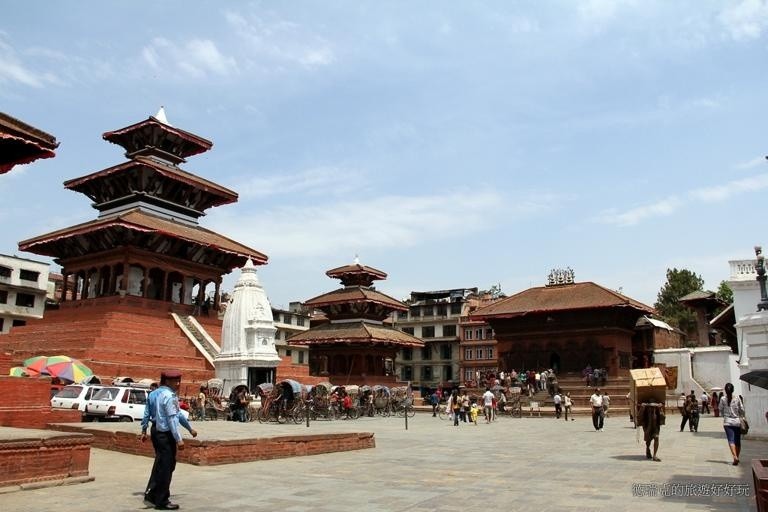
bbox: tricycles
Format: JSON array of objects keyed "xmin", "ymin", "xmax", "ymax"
[
  {"xmin": 490, "ymin": 387, "xmax": 522, "ymax": 417},
  {"xmin": 190, "ymin": 375, "xmax": 415, "ymax": 424}
]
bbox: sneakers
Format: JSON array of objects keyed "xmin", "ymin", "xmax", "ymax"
[{"xmin": 733, "ymin": 458, "xmax": 739, "ymax": 465}]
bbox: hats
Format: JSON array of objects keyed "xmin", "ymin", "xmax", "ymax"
[{"xmin": 161, "ymin": 370, "xmax": 183, "ymax": 379}]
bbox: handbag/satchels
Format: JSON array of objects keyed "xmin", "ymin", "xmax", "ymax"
[{"xmin": 737, "ymin": 396, "xmax": 749, "ymax": 434}]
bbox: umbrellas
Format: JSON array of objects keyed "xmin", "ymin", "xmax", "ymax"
[{"xmin": 8, "ymin": 350, "xmax": 93, "ymax": 387}]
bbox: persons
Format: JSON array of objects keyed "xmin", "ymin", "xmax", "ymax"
[
  {"xmin": 330, "ymin": 391, "xmax": 339, "ymax": 409},
  {"xmin": 154, "ymin": 370, "xmax": 181, "ymax": 510},
  {"xmin": 641, "ymin": 394, "xmax": 661, "ymax": 461},
  {"xmin": 366, "ymin": 391, "xmax": 373, "ymax": 405},
  {"xmin": 720, "ymin": 383, "xmax": 743, "ymax": 465},
  {"xmin": 142, "ymin": 369, "xmax": 197, "ymax": 504},
  {"xmin": 679, "ymin": 383, "xmax": 744, "ymax": 430},
  {"xmin": 430, "ymin": 367, "xmax": 615, "ymax": 431},
  {"xmin": 236, "ymin": 388, "xmax": 249, "ymax": 422},
  {"xmin": 197, "ymin": 386, "xmax": 208, "ymax": 419}
]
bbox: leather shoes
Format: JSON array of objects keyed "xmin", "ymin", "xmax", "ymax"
[
  {"xmin": 145, "ymin": 488, "xmax": 155, "ymax": 505},
  {"xmin": 155, "ymin": 502, "xmax": 179, "ymax": 509}
]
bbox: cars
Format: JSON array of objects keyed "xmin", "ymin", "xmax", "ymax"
[{"xmin": 51, "ymin": 385, "xmax": 85, "ymax": 399}]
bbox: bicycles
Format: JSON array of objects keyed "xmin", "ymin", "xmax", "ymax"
[{"xmin": 438, "ymin": 405, "xmax": 455, "ymax": 420}]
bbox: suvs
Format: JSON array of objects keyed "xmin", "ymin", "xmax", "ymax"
[
  {"xmin": 50, "ymin": 382, "xmax": 112, "ymax": 422},
  {"xmin": 86, "ymin": 382, "xmax": 189, "ymax": 424}
]
